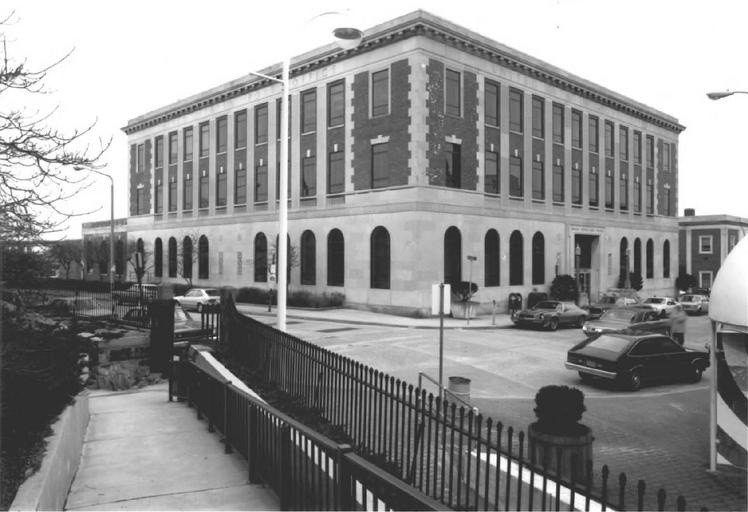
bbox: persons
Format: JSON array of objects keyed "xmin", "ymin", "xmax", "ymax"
[{"xmin": 670, "ymin": 304, "xmax": 687, "ymax": 346}]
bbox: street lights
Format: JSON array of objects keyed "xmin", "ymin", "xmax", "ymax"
[
  {"xmin": 624, "ymin": 246, "xmax": 631, "ymax": 289},
  {"xmin": 267, "ymin": 4, "xmax": 370, "ymax": 387},
  {"xmin": 574, "ymin": 243, "xmax": 581, "ymax": 292},
  {"xmin": 71, "ymin": 165, "xmax": 116, "ymax": 289},
  {"xmin": 704, "ymin": 88, "xmax": 748, "ymax": 102}
]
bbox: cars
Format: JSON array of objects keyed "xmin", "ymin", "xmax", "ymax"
[
  {"xmin": 32, "ymin": 280, "xmax": 221, "ymax": 346},
  {"xmin": 506, "ymin": 290, "xmax": 711, "ymax": 345},
  {"xmin": 561, "ymin": 329, "xmax": 711, "ymax": 392}
]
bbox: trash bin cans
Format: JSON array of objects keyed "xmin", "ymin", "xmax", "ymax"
[{"xmin": 448, "ymin": 377, "xmax": 471, "ymax": 418}]
